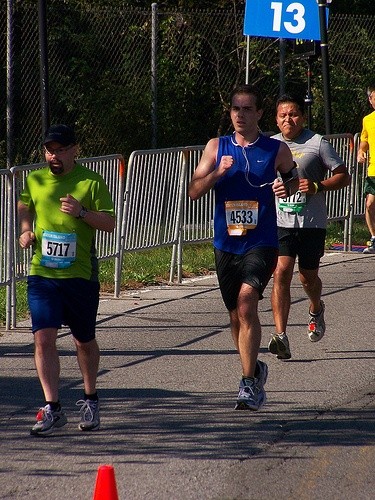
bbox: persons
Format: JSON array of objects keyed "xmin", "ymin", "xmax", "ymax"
[
  {"xmin": 356, "ymin": 85, "xmax": 375, "ymax": 254},
  {"xmin": 266, "ymin": 94, "xmax": 351, "ymax": 360},
  {"xmin": 16, "ymin": 125, "xmax": 116, "ymax": 437},
  {"xmin": 186, "ymin": 86, "xmax": 298, "ymax": 412}
]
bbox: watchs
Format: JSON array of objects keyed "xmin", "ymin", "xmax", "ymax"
[{"xmin": 75, "ymin": 206, "xmax": 88, "ymax": 219}]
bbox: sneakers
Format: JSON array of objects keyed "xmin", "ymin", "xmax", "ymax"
[
  {"xmin": 30, "ymin": 404, "xmax": 68, "ymax": 437},
  {"xmin": 308, "ymin": 299, "xmax": 326, "ymax": 342},
  {"xmin": 268, "ymin": 331, "xmax": 292, "ymax": 359},
  {"xmin": 236, "ymin": 377, "xmax": 266, "ymax": 410},
  {"xmin": 363, "ymin": 237, "xmax": 375, "ymax": 254},
  {"xmin": 76, "ymin": 398, "xmax": 100, "ymax": 432},
  {"xmin": 253, "ymin": 359, "xmax": 269, "ymax": 386}
]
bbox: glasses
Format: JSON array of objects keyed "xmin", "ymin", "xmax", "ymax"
[{"xmin": 43, "ymin": 145, "xmax": 76, "ymax": 155}]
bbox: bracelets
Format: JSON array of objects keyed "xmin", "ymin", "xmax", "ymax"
[{"xmin": 313, "ymin": 181, "xmax": 322, "ymax": 194}]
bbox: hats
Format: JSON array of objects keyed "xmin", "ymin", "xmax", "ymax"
[{"xmin": 43, "ymin": 124, "xmax": 72, "ymax": 143}]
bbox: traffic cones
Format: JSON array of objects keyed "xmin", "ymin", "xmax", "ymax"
[{"xmin": 94, "ymin": 464, "xmax": 120, "ymax": 500}]
